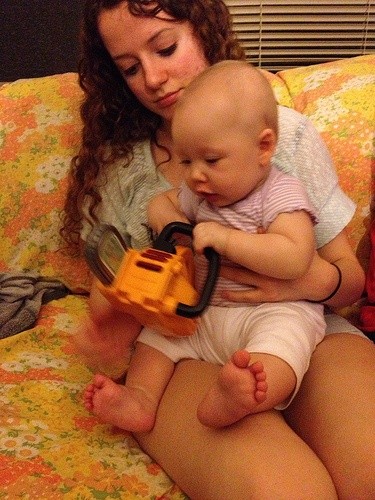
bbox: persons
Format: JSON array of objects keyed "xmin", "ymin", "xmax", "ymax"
[
  {"xmin": 82, "ymin": 57, "xmax": 325, "ymax": 434},
  {"xmin": 61, "ymin": 1, "xmax": 375, "ymax": 500}
]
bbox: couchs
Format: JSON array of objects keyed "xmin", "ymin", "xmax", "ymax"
[{"xmin": 1, "ymin": 53, "xmax": 375, "ymax": 500}]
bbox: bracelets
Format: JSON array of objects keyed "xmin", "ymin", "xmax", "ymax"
[{"xmin": 306, "ymin": 260, "xmax": 343, "ymax": 304}]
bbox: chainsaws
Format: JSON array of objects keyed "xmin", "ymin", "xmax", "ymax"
[{"xmin": 82, "ymin": 221, "xmax": 221, "ymax": 339}]
